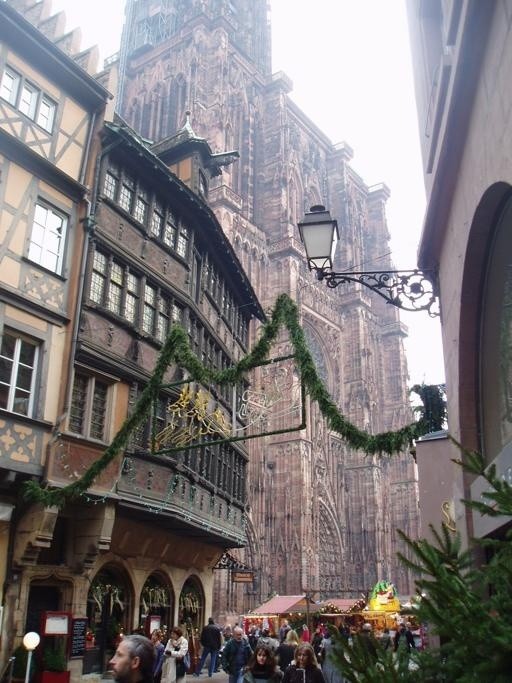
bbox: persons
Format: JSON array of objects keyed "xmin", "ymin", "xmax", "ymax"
[{"xmin": 109, "ymin": 616, "xmax": 417, "ymax": 683}]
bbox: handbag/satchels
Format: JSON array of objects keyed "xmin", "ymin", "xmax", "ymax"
[{"xmin": 181, "ymin": 652, "xmax": 191, "ymax": 670}]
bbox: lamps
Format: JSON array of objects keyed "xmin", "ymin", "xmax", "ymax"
[{"xmin": 298, "ymin": 205, "xmax": 443, "ymax": 324}]
